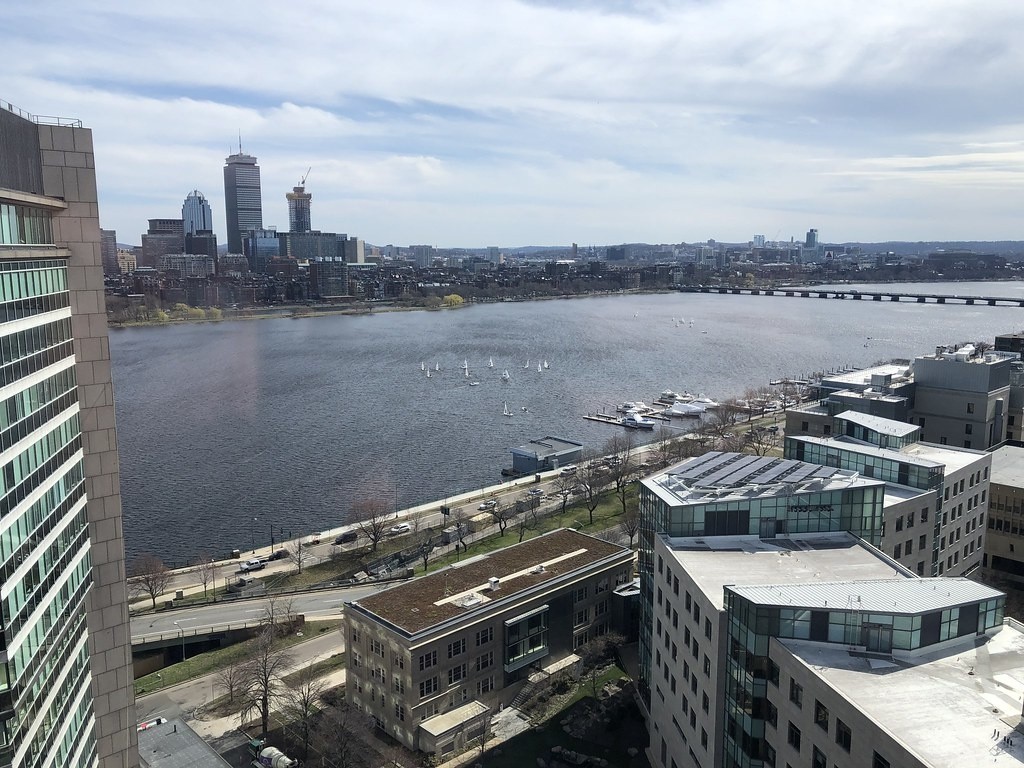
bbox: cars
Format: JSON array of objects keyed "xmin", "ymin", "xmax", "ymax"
[
  {"xmin": 744, "ymin": 425, "xmax": 779, "ymax": 438},
  {"xmin": 527, "ymin": 488, "xmax": 544, "ymax": 496},
  {"xmin": 479, "ymin": 500, "xmax": 498, "ymax": 510},
  {"xmin": 267, "ymin": 548, "xmax": 290, "ymax": 562},
  {"xmin": 556, "ymin": 453, "xmax": 626, "ymax": 499},
  {"xmin": 390, "ymin": 523, "xmax": 411, "ymax": 535}
]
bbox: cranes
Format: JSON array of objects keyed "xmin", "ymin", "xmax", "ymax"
[{"xmin": 301, "ymin": 166, "xmax": 312, "ymax": 188}]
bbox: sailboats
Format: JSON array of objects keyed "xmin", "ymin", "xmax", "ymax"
[
  {"xmin": 419, "ymin": 355, "xmax": 549, "ymax": 386},
  {"xmin": 502, "ymin": 400, "xmax": 515, "ymax": 417}
]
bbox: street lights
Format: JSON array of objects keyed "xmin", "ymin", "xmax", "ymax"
[{"xmin": 173, "ymin": 621, "xmax": 186, "ymax": 662}]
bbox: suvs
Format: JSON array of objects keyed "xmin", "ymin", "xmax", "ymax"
[{"xmin": 335, "ymin": 531, "xmax": 358, "ymax": 545}]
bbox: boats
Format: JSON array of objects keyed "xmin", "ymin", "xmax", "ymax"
[
  {"xmin": 617, "ymin": 387, "xmax": 721, "ymax": 428},
  {"xmin": 728, "ymin": 391, "xmax": 808, "ymax": 413}
]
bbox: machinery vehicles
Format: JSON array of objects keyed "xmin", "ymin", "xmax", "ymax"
[{"xmin": 247, "ymin": 736, "xmax": 299, "ymax": 768}]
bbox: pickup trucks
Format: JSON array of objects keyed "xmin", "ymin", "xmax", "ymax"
[{"xmin": 240, "ymin": 558, "xmax": 269, "ymax": 573}]
